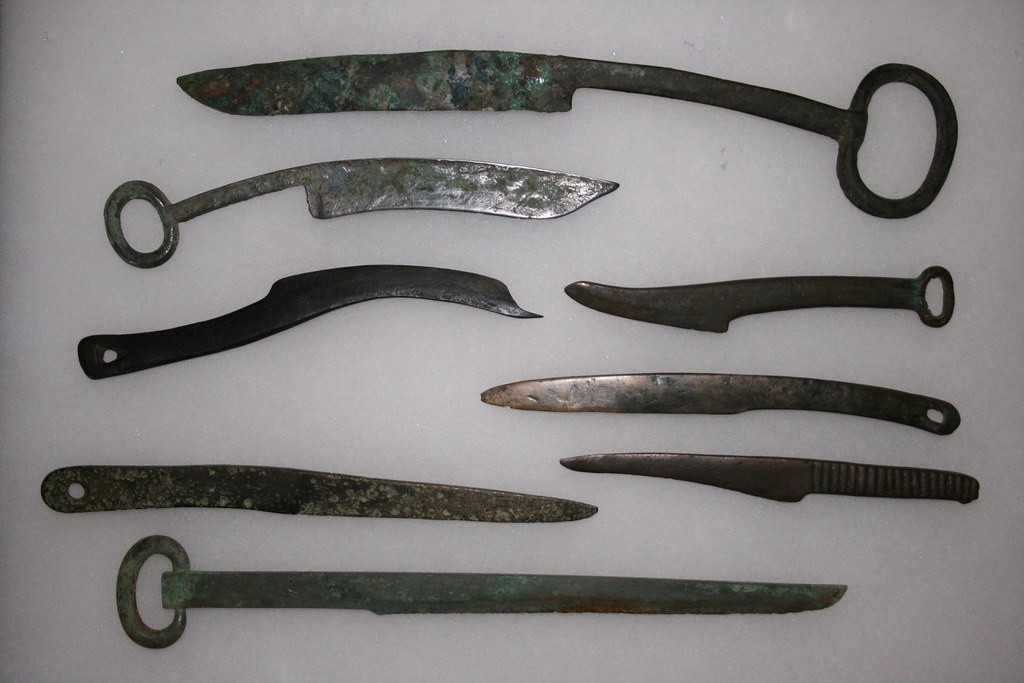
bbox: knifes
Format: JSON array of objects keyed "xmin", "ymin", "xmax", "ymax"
[
  {"xmin": 40, "ymin": 463, "xmax": 597, "ymax": 522},
  {"xmin": 175, "ymin": 51, "xmax": 958, "ymax": 219},
  {"xmin": 105, "ymin": 158, "xmax": 620, "ymax": 271},
  {"xmin": 115, "ymin": 535, "xmax": 847, "ymax": 650},
  {"xmin": 567, "ymin": 264, "xmax": 957, "ymax": 331},
  {"xmin": 79, "ymin": 266, "xmax": 543, "ymax": 381},
  {"xmin": 481, "ymin": 372, "xmax": 961, "ymax": 435},
  {"xmin": 562, "ymin": 455, "xmax": 983, "ymax": 505}
]
